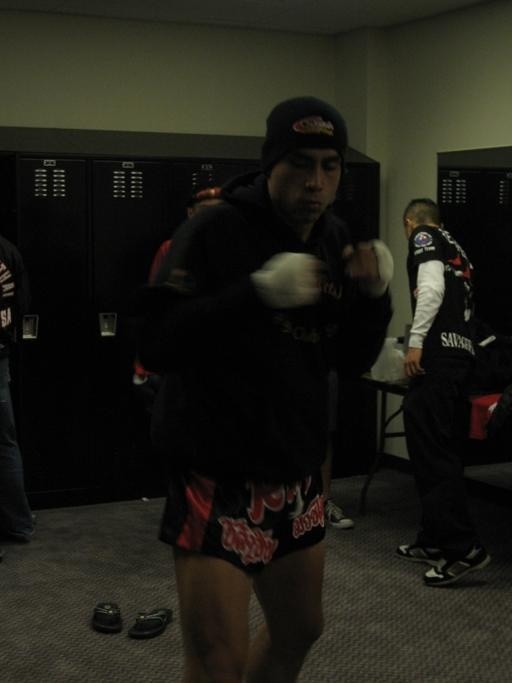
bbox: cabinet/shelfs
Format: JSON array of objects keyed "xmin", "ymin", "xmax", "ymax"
[
  {"xmin": 0, "ymin": 150, "xmax": 380, "ymax": 512},
  {"xmin": 438, "ymin": 168, "xmax": 510, "ymax": 393}
]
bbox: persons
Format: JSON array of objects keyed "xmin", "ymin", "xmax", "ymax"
[
  {"xmin": 0, "ymin": 231, "xmax": 42, "ymax": 545},
  {"xmin": 391, "ymin": 197, "xmax": 497, "ymax": 587},
  {"xmin": 128, "ymin": 92, "xmax": 400, "ymax": 682},
  {"xmin": 132, "ymin": 183, "xmax": 222, "ymax": 451}
]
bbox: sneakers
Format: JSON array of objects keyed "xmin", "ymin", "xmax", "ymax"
[
  {"xmin": 325, "ymin": 498, "xmax": 354, "ymax": 529},
  {"xmin": 394, "ymin": 538, "xmax": 491, "ymax": 586},
  {"xmin": 11, "ymin": 526, "xmax": 37, "ymax": 543}
]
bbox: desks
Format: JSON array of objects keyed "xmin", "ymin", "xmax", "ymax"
[{"xmin": 358, "ymin": 373, "xmax": 410, "ymax": 510}]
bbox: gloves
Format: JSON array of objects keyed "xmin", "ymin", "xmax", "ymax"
[
  {"xmin": 250, "ymin": 252, "xmax": 321, "ymax": 310},
  {"xmin": 362, "ymin": 241, "xmax": 394, "ymax": 298}
]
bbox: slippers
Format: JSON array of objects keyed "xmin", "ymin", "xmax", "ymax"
[
  {"xmin": 128, "ymin": 608, "xmax": 173, "ymax": 638},
  {"xmin": 92, "ymin": 601, "xmax": 122, "ymax": 633}
]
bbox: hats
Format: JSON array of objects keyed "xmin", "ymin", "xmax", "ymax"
[{"xmin": 260, "ymin": 98, "xmax": 347, "ymax": 177}]
